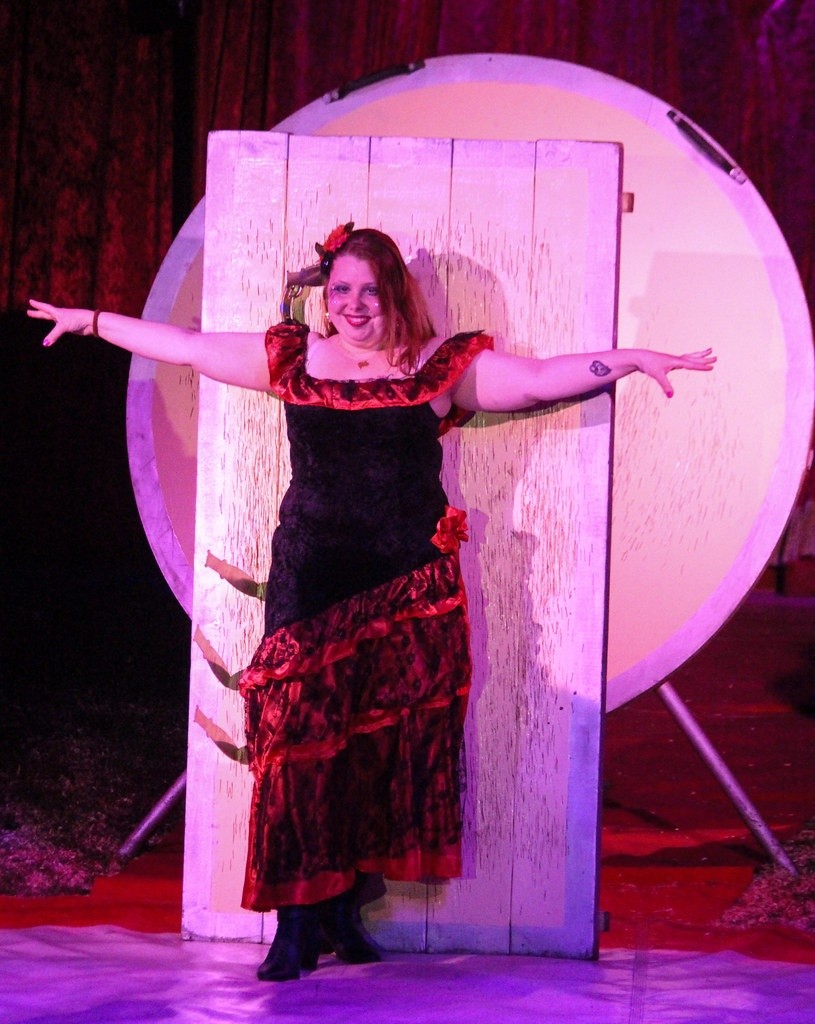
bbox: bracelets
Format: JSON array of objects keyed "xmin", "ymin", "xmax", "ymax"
[{"xmin": 93, "ymin": 309, "xmax": 102, "ymax": 336}]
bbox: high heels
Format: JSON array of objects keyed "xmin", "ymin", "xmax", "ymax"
[
  {"xmin": 257, "ymin": 905, "xmax": 320, "ymax": 981},
  {"xmin": 320, "ymin": 893, "xmax": 375, "ymax": 964}
]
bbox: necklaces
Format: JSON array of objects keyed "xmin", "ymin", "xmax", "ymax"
[{"xmin": 357, "ymin": 360, "xmax": 369, "ymax": 369}]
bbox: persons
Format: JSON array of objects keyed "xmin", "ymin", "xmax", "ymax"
[{"xmin": 27, "ymin": 221, "xmax": 719, "ymax": 984}]
